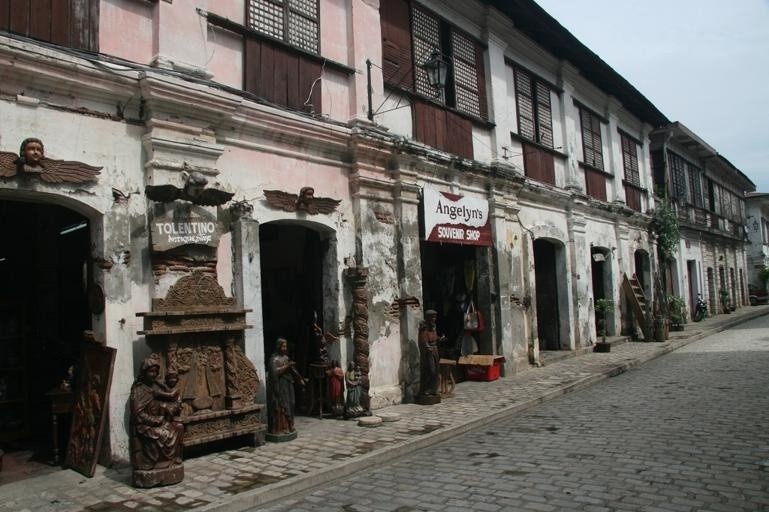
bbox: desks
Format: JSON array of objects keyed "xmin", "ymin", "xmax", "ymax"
[{"xmin": 438, "ymin": 358, "xmax": 457, "ymax": 395}]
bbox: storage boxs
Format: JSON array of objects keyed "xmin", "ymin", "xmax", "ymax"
[{"xmin": 464, "ymin": 354, "xmax": 506, "ymax": 382}]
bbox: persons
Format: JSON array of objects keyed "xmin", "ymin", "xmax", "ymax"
[
  {"xmin": 124, "ymin": 350, "xmax": 186, "ymax": 489},
  {"xmin": 324, "ymin": 359, "xmax": 344, "ymax": 416},
  {"xmin": 14, "ymin": 138, "xmax": 48, "ymax": 176},
  {"xmin": 293, "ymin": 186, "xmax": 316, "ymax": 211},
  {"xmin": 263, "ymin": 335, "xmax": 307, "ymax": 441},
  {"xmin": 417, "ymin": 307, "xmax": 448, "ymax": 406},
  {"xmin": 154, "ymin": 370, "xmax": 183, "ymax": 426},
  {"xmin": 345, "ymin": 362, "xmax": 365, "ymax": 419},
  {"xmin": 182, "ymin": 172, "xmax": 210, "ymax": 203}
]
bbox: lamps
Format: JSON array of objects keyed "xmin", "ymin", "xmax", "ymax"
[{"xmin": 366, "ymin": 42, "xmax": 451, "ymax": 119}]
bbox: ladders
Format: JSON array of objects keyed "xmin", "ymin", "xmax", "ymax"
[{"xmin": 623, "ymin": 272, "xmax": 654, "ymax": 340}]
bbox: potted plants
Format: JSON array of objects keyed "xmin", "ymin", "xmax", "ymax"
[{"xmin": 595, "ymin": 299, "xmax": 613, "ymax": 352}]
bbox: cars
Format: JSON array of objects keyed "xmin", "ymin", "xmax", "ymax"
[{"xmin": 747, "ymin": 283, "xmax": 769, "ymax": 306}]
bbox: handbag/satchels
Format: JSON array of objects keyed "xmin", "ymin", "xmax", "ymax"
[{"xmin": 464, "ymin": 312, "xmax": 478, "ymax": 329}]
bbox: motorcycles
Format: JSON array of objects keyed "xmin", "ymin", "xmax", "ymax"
[{"xmin": 694, "ymin": 294, "xmax": 709, "ymax": 322}]
